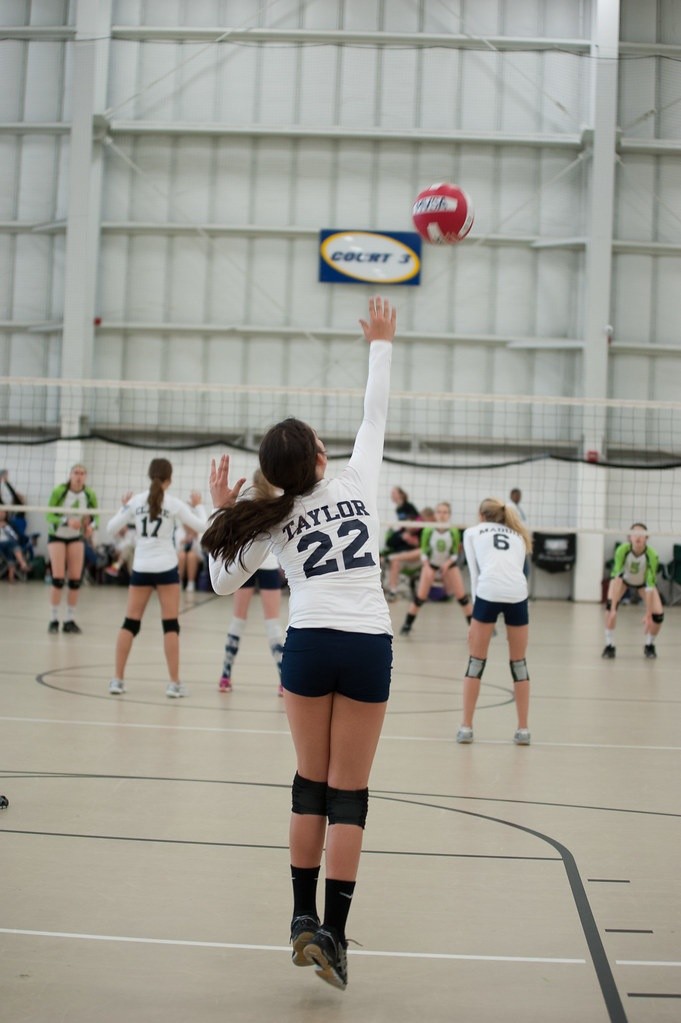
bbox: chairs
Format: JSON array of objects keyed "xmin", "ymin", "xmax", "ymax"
[
  {"xmin": 531, "ymin": 532, "xmax": 576, "ymax": 599},
  {"xmin": 605, "ymin": 542, "xmax": 681, "ymax": 606}
]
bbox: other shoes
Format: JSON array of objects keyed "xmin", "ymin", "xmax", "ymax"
[
  {"xmin": 185, "ymin": 586, "xmax": 195, "ymax": 592},
  {"xmin": 388, "ymin": 592, "xmax": 397, "ymax": 602},
  {"xmin": 105, "ymin": 566, "xmax": 119, "ymax": 577},
  {"xmin": 0, "ymin": 795, "xmax": 9, "ymax": 808},
  {"xmin": 399, "ymin": 625, "xmax": 411, "ymax": 635}
]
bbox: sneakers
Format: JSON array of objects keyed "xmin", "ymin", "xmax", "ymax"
[
  {"xmin": 278, "ymin": 681, "xmax": 286, "ymax": 696},
  {"xmin": 109, "ymin": 679, "xmax": 126, "ymax": 694},
  {"xmin": 644, "ymin": 644, "xmax": 657, "ymax": 659},
  {"xmin": 62, "ymin": 620, "xmax": 82, "ymax": 634},
  {"xmin": 513, "ymin": 727, "xmax": 531, "ymax": 745},
  {"xmin": 303, "ymin": 924, "xmax": 364, "ymax": 991},
  {"xmin": 457, "ymin": 724, "xmax": 473, "ymax": 744},
  {"xmin": 219, "ymin": 677, "xmax": 233, "ymax": 692},
  {"xmin": 166, "ymin": 683, "xmax": 189, "ymax": 698},
  {"xmin": 601, "ymin": 645, "xmax": 615, "ymax": 658},
  {"xmin": 289, "ymin": 915, "xmax": 320, "ymax": 967},
  {"xmin": 47, "ymin": 619, "xmax": 60, "ymax": 633}
]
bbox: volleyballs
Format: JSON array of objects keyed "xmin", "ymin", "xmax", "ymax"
[{"xmin": 415, "ymin": 185, "xmax": 475, "ymax": 243}]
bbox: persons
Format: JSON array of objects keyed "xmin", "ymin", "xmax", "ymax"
[
  {"xmin": 46, "ymin": 464, "xmax": 101, "ymax": 634},
  {"xmin": 508, "ymin": 490, "xmax": 529, "ymax": 582},
  {"xmin": 107, "ymin": 458, "xmax": 208, "ymax": 700},
  {"xmin": 379, "ymin": 485, "xmax": 474, "ymax": 639},
  {"xmin": 455, "ymin": 498, "xmax": 533, "ymax": 745},
  {"xmin": 217, "ymin": 469, "xmax": 284, "ymax": 699},
  {"xmin": 600, "ymin": 522, "xmax": 664, "ymax": 658},
  {"xmin": 0, "ymin": 470, "xmax": 214, "ymax": 594},
  {"xmin": 206, "ymin": 296, "xmax": 397, "ymax": 990}
]
